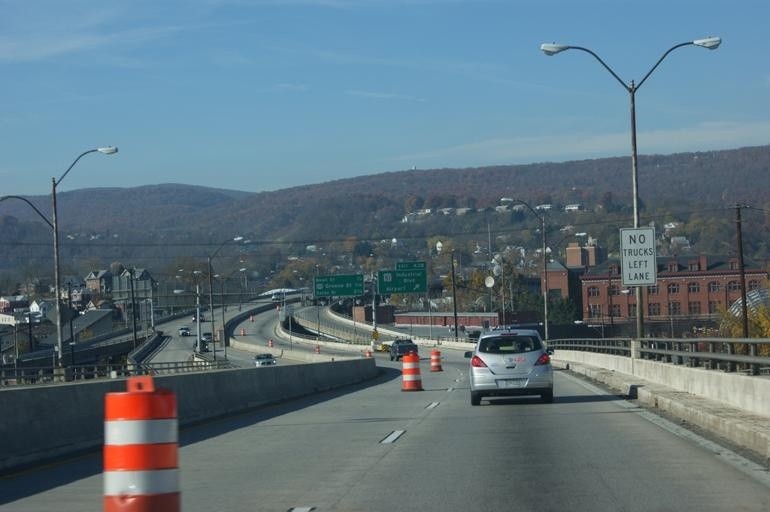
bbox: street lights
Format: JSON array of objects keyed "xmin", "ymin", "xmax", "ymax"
[
  {"xmin": 284, "ymin": 241, "xmax": 342, "ymax": 340},
  {"xmin": 1, "ymin": 144, "xmax": 120, "ymax": 384},
  {"xmin": 537, "ymin": 32, "xmax": 725, "ymax": 338},
  {"xmin": 164, "ymin": 232, "xmax": 251, "ymax": 361},
  {"xmin": 499, "ymin": 195, "xmax": 550, "ymax": 341}
]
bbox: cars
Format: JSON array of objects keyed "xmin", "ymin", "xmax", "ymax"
[
  {"xmin": 463, "ymin": 327, "xmax": 557, "ymax": 407},
  {"xmin": 252, "ymin": 352, "xmax": 277, "ymax": 368}
]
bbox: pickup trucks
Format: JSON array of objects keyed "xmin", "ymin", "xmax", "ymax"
[{"xmin": 388, "ymin": 337, "xmax": 419, "ymax": 362}]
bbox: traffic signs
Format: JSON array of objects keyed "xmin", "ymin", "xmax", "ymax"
[
  {"xmin": 314, "ymin": 275, "xmax": 364, "ymax": 297},
  {"xmin": 395, "ymin": 261, "xmax": 426, "ymax": 271},
  {"xmin": 377, "ymin": 271, "xmax": 427, "ymax": 296}
]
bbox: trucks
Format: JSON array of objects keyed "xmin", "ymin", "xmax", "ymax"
[{"xmin": 271, "ymin": 291, "xmax": 285, "ymax": 301}]
